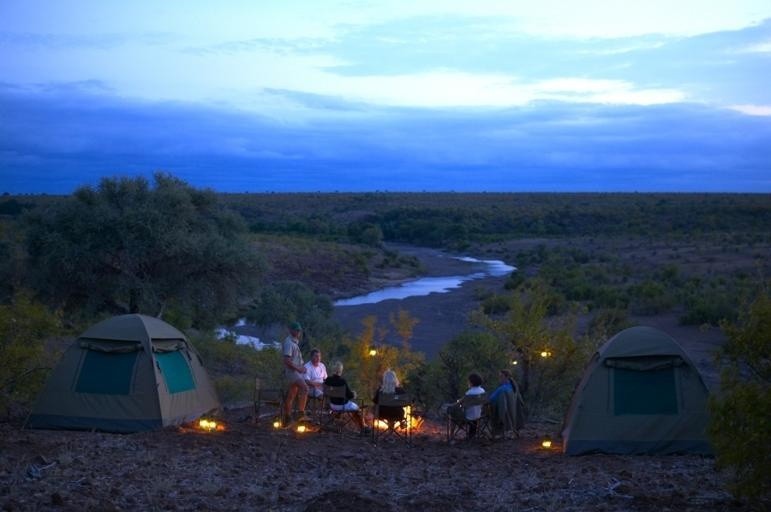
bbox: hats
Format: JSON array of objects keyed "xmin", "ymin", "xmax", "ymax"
[{"xmin": 287, "ymin": 322, "xmax": 301, "ymax": 330}]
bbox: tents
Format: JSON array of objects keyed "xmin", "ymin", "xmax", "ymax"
[
  {"xmin": 21, "ymin": 312, "xmax": 223, "ymax": 436},
  {"xmin": 560, "ymin": 324, "xmax": 723, "ymax": 460}
]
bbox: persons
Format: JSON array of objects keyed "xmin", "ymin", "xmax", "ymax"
[
  {"xmin": 372, "ymin": 367, "xmax": 409, "ymax": 442},
  {"xmin": 443, "ymin": 372, "xmax": 485, "ymax": 442},
  {"xmin": 302, "ymin": 348, "xmax": 342, "ymax": 426},
  {"xmin": 321, "ymin": 359, "xmax": 372, "ymax": 434},
  {"xmin": 281, "ymin": 319, "xmax": 313, "ymax": 426},
  {"xmin": 483, "ymin": 367, "xmax": 517, "ymax": 441}
]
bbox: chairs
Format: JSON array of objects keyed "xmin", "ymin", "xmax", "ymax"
[
  {"xmin": 484, "ymin": 389, "xmax": 525, "ymax": 442},
  {"xmin": 319, "ymin": 383, "xmax": 365, "ymax": 437},
  {"xmin": 291, "ymin": 373, "xmax": 323, "ymax": 420},
  {"xmin": 370, "ymin": 390, "xmax": 415, "ymax": 450},
  {"xmin": 252, "ymin": 375, "xmax": 289, "ymax": 429},
  {"xmin": 445, "ymin": 391, "xmax": 496, "ymax": 451}
]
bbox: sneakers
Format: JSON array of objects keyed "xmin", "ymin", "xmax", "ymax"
[
  {"xmin": 400, "ymin": 423, "xmax": 407, "ymax": 430},
  {"xmin": 364, "ymin": 426, "xmax": 372, "ymax": 433},
  {"xmin": 297, "ymin": 416, "xmax": 313, "ymax": 422}
]
